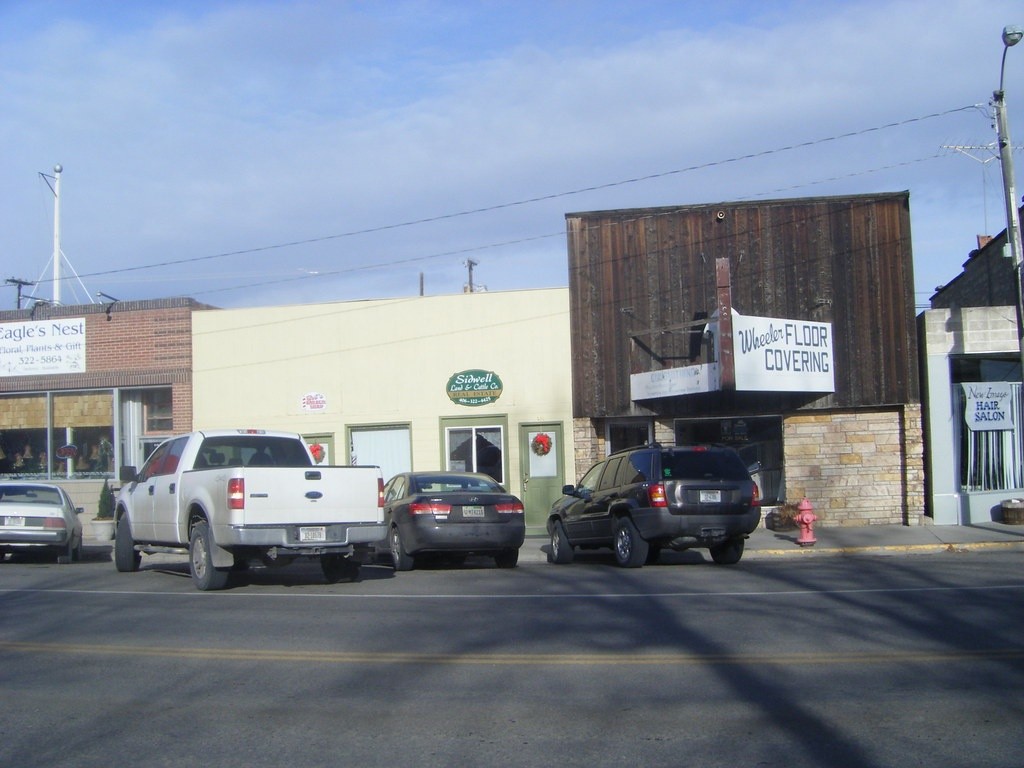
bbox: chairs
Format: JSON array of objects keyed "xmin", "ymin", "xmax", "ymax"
[
  {"xmin": 246, "ymin": 452, "xmax": 273, "ymax": 466},
  {"xmin": 206, "ymin": 452, "xmax": 225, "ymax": 466}
]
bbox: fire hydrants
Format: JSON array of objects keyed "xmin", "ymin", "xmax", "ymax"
[{"xmin": 792, "ymin": 495, "xmax": 819, "ymax": 546}]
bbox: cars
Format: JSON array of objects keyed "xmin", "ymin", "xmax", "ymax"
[
  {"xmin": 0, "ymin": 482, "xmax": 84, "ymax": 565},
  {"xmin": 371, "ymin": 470, "xmax": 527, "ymax": 570}
]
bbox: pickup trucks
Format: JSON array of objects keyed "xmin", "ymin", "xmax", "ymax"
[{"xmin": 110, "ymin": 427, "xmax": 392, "ymax": 593}]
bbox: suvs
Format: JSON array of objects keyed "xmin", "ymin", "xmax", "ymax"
[{"xmin": 545, "ymin": 440, "xmax": 762, "ymax": 567}]
